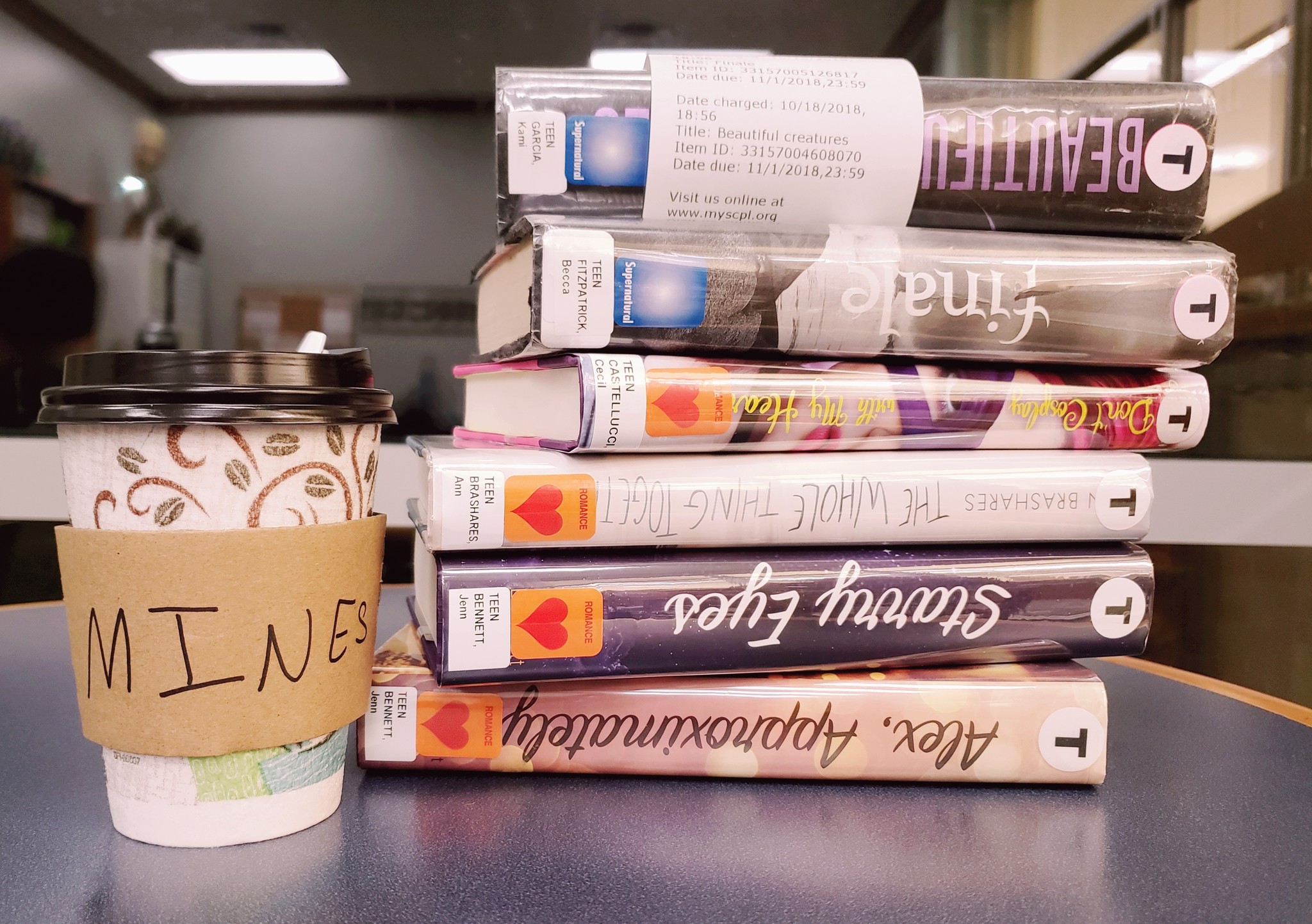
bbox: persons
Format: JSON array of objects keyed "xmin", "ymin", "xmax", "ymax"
[{"xmin": 0, "ymin": 113, "xmax": 107, "ymax": 427}]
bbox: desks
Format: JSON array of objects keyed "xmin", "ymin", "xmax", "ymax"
[
  {"xmin": 0, "ymin": 435, "xmax": 417, "ymax": 604},
  {"xmin": 1, "ymin": 598, "xmax": 1312, "ymax": 924}
]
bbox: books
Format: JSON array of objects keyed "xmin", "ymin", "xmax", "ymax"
[
  {"xmin": 354, "ymin": 618, "xmax": 1109, "ymax": 788},
  {"xmin": 402, "ymin": 497, "xmax": 1156, "ymax": 689},
  {"xmin": 468, "ymin": 213, "xmax": 1241, "ymax": 369},
  {"xmin": 449, "ymin": 352, "xmax": 1212, "ymax": 458},
  {"xmin": 404, "ymin": 433, "xmax": 1156, "ymax": 553},
  {"xmin": 492, "ymin": 65, "xmax": 1219, "ymax": 240}
]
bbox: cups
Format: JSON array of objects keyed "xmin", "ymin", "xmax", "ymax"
[{"xmin": 38, "ymin": 346, "xmax": 400, "ymax": 848}]
